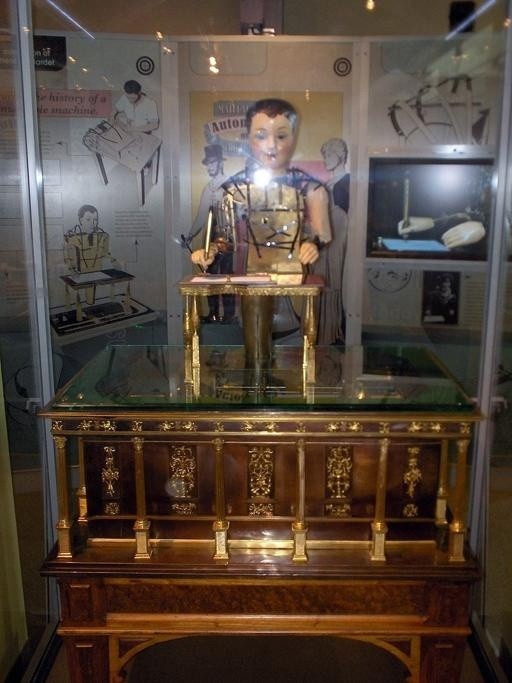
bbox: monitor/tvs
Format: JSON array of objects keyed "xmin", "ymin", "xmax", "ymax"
[{"xmin": 366, "ymin": 158, "xmax": 493, "ymax": 261}]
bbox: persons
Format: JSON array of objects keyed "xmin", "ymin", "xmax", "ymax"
[
  {"xmin": 396, "ymin": 164, "xmax": 492, "ymax": 249},
  {"xmin": 421, "ymin": 271, "xmax": 459, "ymax": 326},
  {"xmin": 175, "ymin": 139, "xmax": 240, "ymax": 324},
  {"xmin": 315, "ymin": 135, "xmax": 351, "ymax": 349},
  {"xmin": 103, "ymin": 76, "xmax": 162, "ymax": 136},
  {"xmin": 185, "ymin": 97, "xmax": 336, "ymax": 274},
  {"xmin": 62, "ymin": 205, "xmax": 122, "ymax": 276}
]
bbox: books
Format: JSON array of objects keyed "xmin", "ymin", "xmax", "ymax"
[{"xmin": 188, "ymin": 274, "xmax": 271, "ymax": 283}]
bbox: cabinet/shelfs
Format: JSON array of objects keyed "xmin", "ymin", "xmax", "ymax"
[{"xmin": 36, "ymin": 339, "xmax": 485, "ymax": 683}]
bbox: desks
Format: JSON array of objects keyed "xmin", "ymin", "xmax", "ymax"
[
  {"xmin": 82, "ymin": 120, "xmax": 165, "ymax": 205},
  {"xmin": 179, "ymin": 272, "xmax": 323, "ymax": 395},
  {"xmin": 59, "ymin": 268, "xmax": 137, "ymax": 319}
]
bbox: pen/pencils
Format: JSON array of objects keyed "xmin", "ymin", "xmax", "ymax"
[
  {"xmin": 404, "ymin": 178, "xmax": 410, "ymax": 240},
  {"xmin": 203, "ymin": 206, "xmax": 213, "ymax": 274},
  {"xmin": 107, "ymin": 347, "xmax": 115, "ymax": 375}
]
